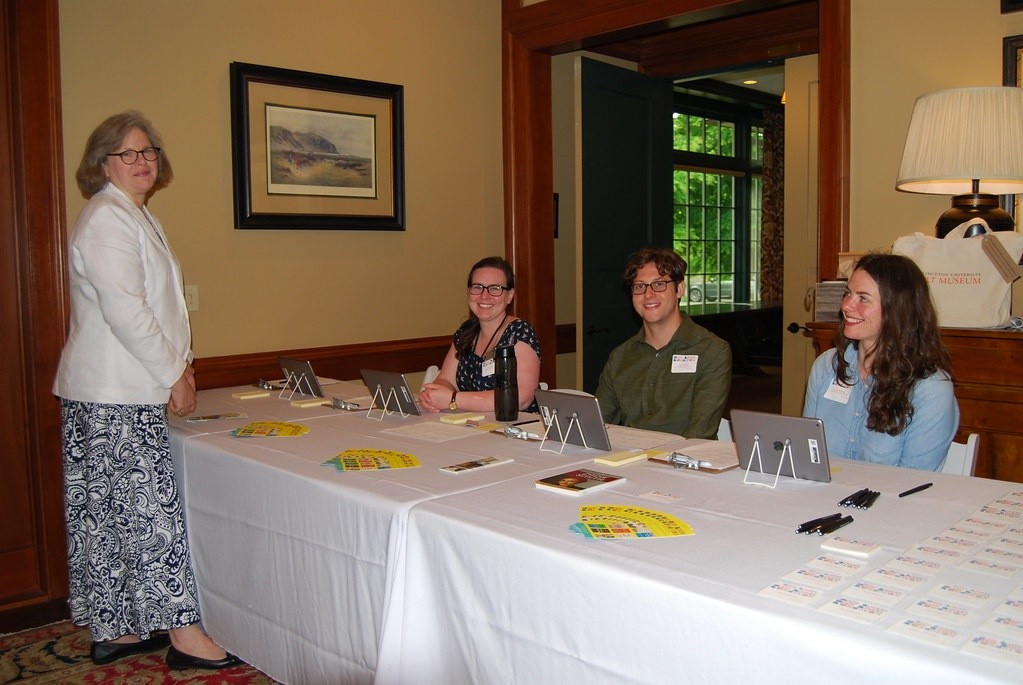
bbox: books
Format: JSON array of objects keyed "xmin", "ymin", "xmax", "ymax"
[{"xmin": 535, "ymin": 468, "xmax": 627, "ymax": 497}]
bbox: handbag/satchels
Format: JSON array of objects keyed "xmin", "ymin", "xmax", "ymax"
[{"xmin": 892, "ymin": 217, "xmax": 1023, "ymax": 329}]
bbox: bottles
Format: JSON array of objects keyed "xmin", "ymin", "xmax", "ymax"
[{"xmin": 493, "ymin": 337, "xmax": 520, "ymax": 422}]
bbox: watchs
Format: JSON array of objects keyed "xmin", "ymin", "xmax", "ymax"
[{"xmin": 448, "ymin": 390, "xmax": 457, "ymax": 411}]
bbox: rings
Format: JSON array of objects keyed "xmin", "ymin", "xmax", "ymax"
[{"xmin": 181, "ymin": 411, "xmax": 183, "ymax": 413}]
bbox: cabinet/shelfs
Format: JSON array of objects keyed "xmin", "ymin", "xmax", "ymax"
[{"xmin": 807, "ymin": 321, "xmax": 1022, "ymax": 484}]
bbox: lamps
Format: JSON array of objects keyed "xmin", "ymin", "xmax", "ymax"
[{"xmin": 895, "ymin": 86, "xmax": 1023, "ymax": 327}]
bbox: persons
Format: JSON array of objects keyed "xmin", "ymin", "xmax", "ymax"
[
  {"xmin": 802, "ymin": 252, "xmax": 960, "ymax": 473},
  {"xmin": 418, "ymin": 255, "xmax": 542, "ymax": 414},
  {"xmin": 51, "ymin": 107, "xmax": 247, "ymax": 671},
  {"xmin": 595, "ymin": 243, "xmax": 733, "ymax": 440},
  {"xmin": 559, "ymin": 477, "xmax": 587, "ymax": 488}
]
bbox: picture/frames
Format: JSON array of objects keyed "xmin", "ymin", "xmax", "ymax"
[
  {"xmin": 1002, "ymin": 35, "xmax": 1023, "ymax": 220},
  {"xmin": 228, "ymin": 61, "xmax": 406, "ymax": 230}
]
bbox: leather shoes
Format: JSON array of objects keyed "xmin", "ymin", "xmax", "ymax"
[
  {"xmin": 166, "ymin": 645, "xmax": 245, "ymax": 670},
  {"xmin": 90, "ymin": 632, "xmax": 171, "ymax": 665}
]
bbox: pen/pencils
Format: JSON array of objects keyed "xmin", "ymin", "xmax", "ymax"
[
  {"xmin": 795, "ymin": 513, "xmax": 854, "ymax": 536},
  {"xmin": 188, "ymin": 413, "xmax": 240, "ymax": 421},
  {"xmin": 899, "ymin": 483, "xmax": 932, "ymax": 498},
  {"xmin": 837, "ymin": 488, "xmax": 881, "ymax": 510}
]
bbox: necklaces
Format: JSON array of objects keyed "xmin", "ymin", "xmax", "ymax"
[{"xmin": 467, "ymin": 312, "xmax": 508, "ymax": 390}]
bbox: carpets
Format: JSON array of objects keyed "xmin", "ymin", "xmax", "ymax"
[{"xmin": 1, "ymin": 620, "xmax": 284, "ymax": 685}]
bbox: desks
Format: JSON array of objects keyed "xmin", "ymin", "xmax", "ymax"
[
  {"xmin": 676, "ymin": 301, "xmax": 784, "ymax": 369},
  {"xmin": 167, "ymin": 375, "xmax": 1023, "ymax": 684}
]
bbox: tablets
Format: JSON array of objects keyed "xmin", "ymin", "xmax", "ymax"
[
  {"xmin": 730, "ymin": 409, "xmax": 831, "ymax": 483},
  {"xmin": 360, "ymin": 369, "xmax": 421, "ymax": 416},
  {"xmin": 534, "ymin": 389, "xmax": 611, "ymax": 451},
  {"xmin": 277, "ymin": 357, "xmax": 325, "ymax": 397}
]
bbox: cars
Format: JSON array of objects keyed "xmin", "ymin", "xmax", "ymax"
[{"xmin": 690, "ymin": 275, "xmax": 757, "ymax": 302}]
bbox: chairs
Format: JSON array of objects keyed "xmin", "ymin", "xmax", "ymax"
[{"xmin": 939, "ymin": 433, "xmax": 979, "ymax": 476}]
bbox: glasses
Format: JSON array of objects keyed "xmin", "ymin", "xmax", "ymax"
[
  {"xmin": 106, "ymin": 146, "xmax": 161, "ymax": 165},
  {"xmin": 468, "ymin": 283, "xmax": 511, "ymax": 297},
  {"xmin": 630, "ymin": 280, "xmax": 674, "ymax": 295}
]
